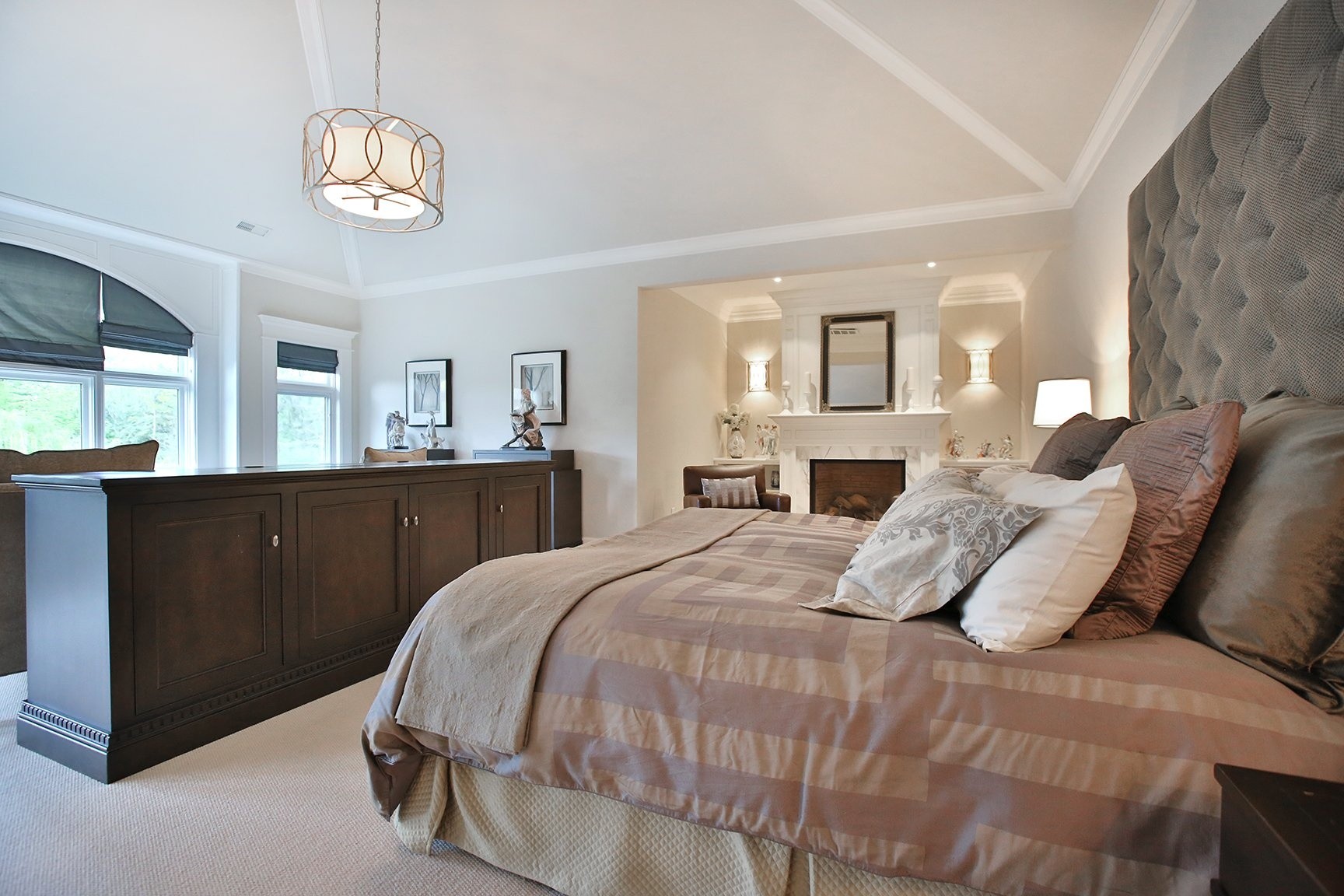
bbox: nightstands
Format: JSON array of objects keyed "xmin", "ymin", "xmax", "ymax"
[{"xmin": 1213, "ymin": 762, "xmax": 1343, "ymax": 896}]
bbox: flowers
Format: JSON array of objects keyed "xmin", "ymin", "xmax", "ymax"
[{"xmin": 718, "ymin": 401, "xmax": 750, "ymax": 427}]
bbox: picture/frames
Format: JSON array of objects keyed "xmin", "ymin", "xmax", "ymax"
[
  {"xmin": 405, "ymin": 358, "xmax": 453, "ymax": 429},
  {"xmin": 509, "ymin": 349, "xmax": 568, "ymax": 425}
]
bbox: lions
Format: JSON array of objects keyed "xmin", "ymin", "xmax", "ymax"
[{"xmin": 387, "ymin": 420, "xmax": 405, "ymax": 447}]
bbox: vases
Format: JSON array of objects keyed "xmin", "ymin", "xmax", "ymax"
[{"xmin": 728, "ymin": 428, "xmax": 746, "ymax": 460}]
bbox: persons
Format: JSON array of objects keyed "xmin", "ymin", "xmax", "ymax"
[
  {"xmin": 950, "ymin": 430, "xmax": 1014, "ymax": 458},
  {"xmin": 756, "ymin": 424, "xmax": 778, "ymax": 455},
  {"xmin": 502, "ymin": 388, "xmax": 543, "ymax": 447},
  {"xmin": 426, "ymin": 411, "xmax": 438, "ymax": 448},
  {"xmin": 386, "ymin": 411, "xmax": 405, "ymax": 446}
]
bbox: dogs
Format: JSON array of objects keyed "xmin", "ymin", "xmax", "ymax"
[
  {"xmin": 424, "ymin": 433, "xmax": 445, "ymax": 448},
  {"xmin": 421, "ymin": 433, "xmax": 429, "ymax": 447}
]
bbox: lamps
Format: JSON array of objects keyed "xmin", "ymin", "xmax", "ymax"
[
  {"xmin": 1032, "ymin": 377, "xmax": 1092, "ymax": 428},
  {"xmin": 965, "ymin": 348, "xmax": 996, "ymax": 386},
  {"xmin": 744, "ymin": 360, "xmax": 769, "ymax": 391},
  {"xmin": 298, "ymin": 2, "xmax": 447, "ymax": 234}
]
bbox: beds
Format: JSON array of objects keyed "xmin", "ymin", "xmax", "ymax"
[{"xmin": 364, "ymin": 2, "xmax": 1343, "ymax": 896}]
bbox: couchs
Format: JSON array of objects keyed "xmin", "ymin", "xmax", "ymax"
[{"xmin": 683, "ymin": 465, "xmax": 791, "ymax": 514}]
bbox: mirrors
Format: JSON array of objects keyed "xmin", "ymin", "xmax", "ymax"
[{"xmin": 820, "ymin": 310, "xmax": 892, "ymax": 413}]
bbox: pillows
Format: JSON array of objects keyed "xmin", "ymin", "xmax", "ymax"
[
  {"xmin": 703, "ymin": 474, "xmax": 760, "ymax": 510},
  {"xmin": 815, "ymin": 464, "xmax": 1037, "ymax": 621},
  {"xmin": 1183, "ymin": 386, "xmax": 1344, "ymax": 717},
  {"xmin": 359, "ymin": 446, "xmax": 429, "ymax": 463},
  {"xmin": 972, "ymin": 457, "xmax": 1022, "ymax": 496},
  {"xmin": 1027, "ymin": 413, "xmax": 1132, "ymax": 480},
  {"xmin": 957, "ymin": 465, "xmax": 1137, "ymax": 652},
  {"xmin": 1082, "ymin": 394, "xmax": 1244, "ymax": 645},
  {"xmin": 1140, "ymin": 388, "xmax": 1203, "ymax": 414},
  {"xmin": 0, "ymin": 439, "xmax": 159, "ymax": 483}
]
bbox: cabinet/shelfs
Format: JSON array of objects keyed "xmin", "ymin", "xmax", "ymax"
[
  {"xmin": 491, "ymin": 461, "xmax": 556, "ymax": 561},
  {"xmin": 104, "ymin": 476, "xmax": 285, "ymax": 785},
  {"xmin": 289, "ymin": 465, "xmax": 487, "ymax": 716}
]
